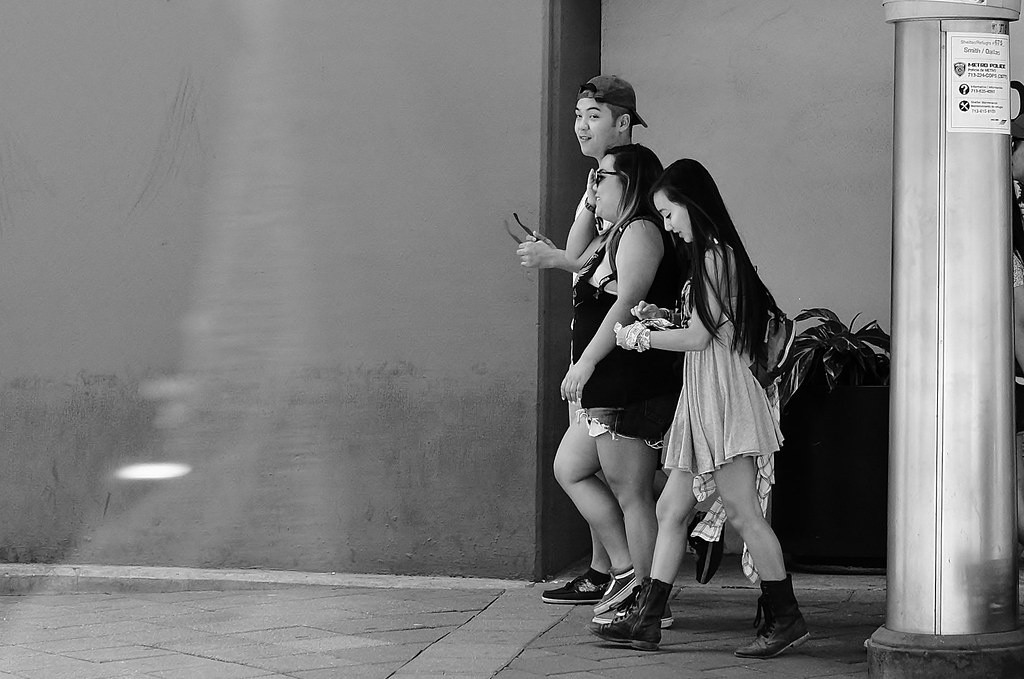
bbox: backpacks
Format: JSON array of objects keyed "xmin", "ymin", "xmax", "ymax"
[{"xmin": 717, "ymin": 240, "xmax": 797, "ymax": 388}]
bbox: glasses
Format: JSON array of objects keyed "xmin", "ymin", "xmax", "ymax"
[
  {"xmin": 504, "ymin": 212, "xmax": 541, "ymax": 244},
  {"xmin": 594, "ymin": 170, "xmax": 625, "ymax": 187}
]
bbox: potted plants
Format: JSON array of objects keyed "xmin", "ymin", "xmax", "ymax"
[{"xmin": 770, "ymin": 308, "xmax": 889, "ymax": 575}]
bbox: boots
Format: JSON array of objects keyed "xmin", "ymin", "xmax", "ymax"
[
  {"xmin": 585, "ymin": 576, "xmax": 673, "ymax": 652},
  {"xmin": 733, "ymin": 572, "xmax": 810, "ymax": 659}
]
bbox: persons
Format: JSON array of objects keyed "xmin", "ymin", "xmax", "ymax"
[
  {"xmin": 588, "ymin": 158, "xmax": 811, "ymax": 659},
  {"xmin": 517, "ymin": 76, "xmax": 726, "ymax": 605},
  {"xmin": 553, "ymin": 143, "xmax": 690, "ymax": 629}
]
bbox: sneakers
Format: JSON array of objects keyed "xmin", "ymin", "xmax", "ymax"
[
  {"xmin": 685, "ymin": 510, "xmax": 725, "ymax": 584},
  {"xmin": 591, "ymin": 599, "xmax": 673, "ymax": 628},
  {"xmin": 542, "ymin": 574, "xmax": 608, "ymax": 604},
  {"xmin": 593, "ymin": 566, "xmax": 638, "ymax": 616}
]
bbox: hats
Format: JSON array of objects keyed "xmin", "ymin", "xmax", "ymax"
[{"xmin": 576, "ymin": 74, "xmax": 648, "ymax": 128}]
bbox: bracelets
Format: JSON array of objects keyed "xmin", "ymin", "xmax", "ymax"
[
  {"xmin": 625, "ymin": 320, "xmax": 651, "ymax": 353},
  {"xmin": 585, "ymin": 197, "xmax": 595, "ymax": 213},
  {"xmin": 659, "ymin": 308, "xmax": 674, "ymax": 324}
]
